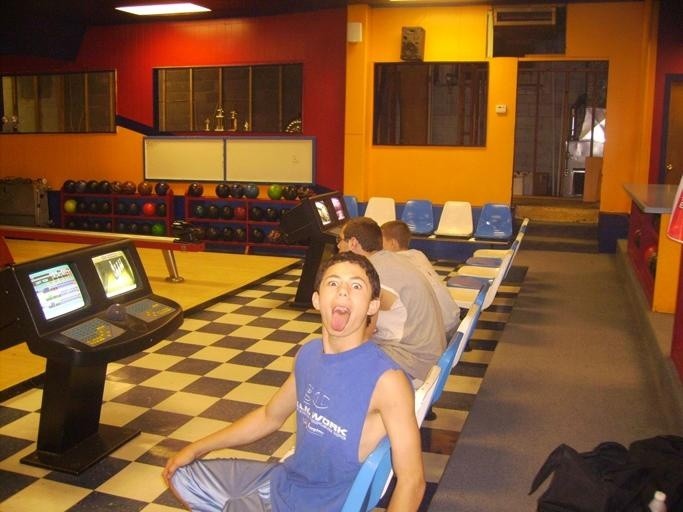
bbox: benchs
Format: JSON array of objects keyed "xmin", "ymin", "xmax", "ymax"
[
  {"xmin": 340, "ymin": 284, "xmax": 487, "ymax": 510},
  {"xmin": 445, "ymin": 218, "xmax": 528, "ymax": 319}
]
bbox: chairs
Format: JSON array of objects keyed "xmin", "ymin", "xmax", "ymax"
[{"xmin": 345, "ymin": 194, "xmax": 514, "ymax": 256}]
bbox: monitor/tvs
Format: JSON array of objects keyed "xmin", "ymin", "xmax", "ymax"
[
  {"xmin": 314, "ymin": 199, "xmax": 332, "ymax": 225},
  {"xmin": 330, "ymin": 195, "xmax": 347, "ymax": 221},
  {"xmin": 89, "ymin": 247, "xmax": 140, "ymax": 301},
  {"xmin": 28, "ymin": 263, "xmax": 87, "ymax": 322}
]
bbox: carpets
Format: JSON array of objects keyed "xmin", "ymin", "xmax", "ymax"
[{"xmin": 1, "ymin": 263, "xmax": 528, "ymax": 510}]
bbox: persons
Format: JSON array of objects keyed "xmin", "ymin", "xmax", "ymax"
[
  {"xmin": 379, "ymin": 221, "xmax": 464, "ymax": 342},
  {"xmin": 333, "ymin": 217, "xmax": 447, "ymax": 389},
  {"xmin": 161, "ymin": 250, "xmax": 428, "ymax": 512}
]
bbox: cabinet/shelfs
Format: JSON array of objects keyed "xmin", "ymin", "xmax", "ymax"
[
  {"xmin": 57, "ymin": 185, "xmax": 319, "ymax": 254},
  {"xmin": 623, "ymin": 188, "xmax": 681, "ymax": 316}
]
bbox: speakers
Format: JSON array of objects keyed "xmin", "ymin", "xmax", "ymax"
[{"xmin": 401, "ymin": 26, "xmax": 425, "ymax": 64}]
